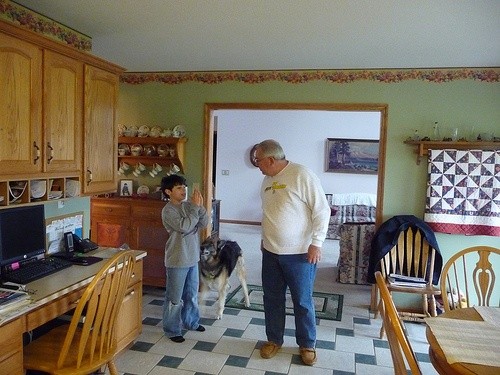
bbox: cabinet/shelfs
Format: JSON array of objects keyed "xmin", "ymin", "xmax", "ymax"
[{"xmin": 0, "ymin": 20, "xmax": 206, "ymax": 375}]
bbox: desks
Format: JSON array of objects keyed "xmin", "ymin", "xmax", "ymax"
[{"xmin": 424, "ymin": 306, "xmax": 500, "ymax": 375}]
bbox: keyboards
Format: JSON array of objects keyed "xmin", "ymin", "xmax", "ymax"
[{"xmin": 5, "ymin": 260, "xmax": 63, "ymax": 284}]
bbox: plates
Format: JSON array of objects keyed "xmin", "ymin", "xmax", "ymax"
[
  {"xmin": 175, "ymin": 125, "xmax": 186, "ymax": 137},
  {"xmin": 118, "ymin": 124, "xmax": 161, "ymax": 138},
  {"xmin": 136, "ymin": 185, "xmax": 150, "ymax": 196},
  {"xmin": 119, "ymin": 144, "xmax": 169, "ymax": 156}
]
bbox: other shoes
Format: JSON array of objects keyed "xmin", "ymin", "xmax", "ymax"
[
  {"xmin": 260, "ymin": 340, "xmax": 283, "ymax": 358},
  {"xmin": 170, "ymin": 335, "xmax": 185, "ymax": 343},
  {"xmin": 196, "ymin": 325, "xmax": 206, "ymax": 332},
  {"xmin": 297, "ymin": 346, "xmax": 317, "ymax": 366}
]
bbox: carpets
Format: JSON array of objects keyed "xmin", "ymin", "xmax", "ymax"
[{"xmin": 225, "ymin": 282, "xmax": 345, "ymax": 322}]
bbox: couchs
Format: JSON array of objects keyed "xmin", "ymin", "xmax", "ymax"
[
  {"xmin": 321, "ymin": 193, "xmax": 377, "ymax": 240},
  {"xmin": 337, "ymin": 222, "xmax": 375, "ymax": 286}
]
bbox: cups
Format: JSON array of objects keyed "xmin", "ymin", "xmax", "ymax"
[
  {"xmin": 119, "ymin": 129, "xmax": 181, "ymax": 137},
  {"xmin": 149, "ymin": 162, "xmax": 163, "ymax": 177},
  {"xmin": 133, "ymin": 162, "xmax": 146, "ymax": 177},
  {"xmin": 167, "ymin": 163, "xmax": 180, "ymax": 175},
  {"xmin": 119, "ymin": 149, "xmax": 175, "ymax": 157},
  {"xmin": 118, "ymin": 162, "xmax": 129, "ymax": 173},
  {"xmin": 451, "ymin": 127, "xmax": 460, "ymax": 142}
]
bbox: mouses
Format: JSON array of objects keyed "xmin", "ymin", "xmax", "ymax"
[{"xmin": 75, "ymin": 258, "xmax": 89, "ymax": 263}]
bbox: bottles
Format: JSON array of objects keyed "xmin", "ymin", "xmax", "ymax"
[
  {"xmin": 431, "ymin": 122, "xmax": 440, "ymax": 140},
  {"xmin": 413, "ymin": 129, "xmax": 419, "ymax": 141}
]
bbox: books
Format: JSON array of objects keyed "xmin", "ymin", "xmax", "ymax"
[{"xmin": 0, "ymin": 288, "xmax": 33, "ymax": 315}]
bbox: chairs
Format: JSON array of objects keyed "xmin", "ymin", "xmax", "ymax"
[
  {"xmin": 369, "ymin": 215, "xmax": 500, "ymax": 375},
  {"xmin": 23, "ymin": 250, "xmax": 136, "ymax": 375}
]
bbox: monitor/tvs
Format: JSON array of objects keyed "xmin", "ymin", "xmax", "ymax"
[{"xmin": 0, "ymin": 204, "xmax": 47, "ymax": 271}]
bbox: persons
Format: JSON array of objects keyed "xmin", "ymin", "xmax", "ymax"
[
  {"xmin": 161, "ymin": 174, "xmax": 208, "ymax": 343},
  {"xmin": 255, "ymin": 139, "xmax": 330, "ymax": 365},
  {"xmin": 122, "ymin": 183, "xmax": 130, "ymax": 196}
]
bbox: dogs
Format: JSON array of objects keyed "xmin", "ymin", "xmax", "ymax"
[{"xmin": 198, "ymin": 233, "xmax": 251, "ymax": 320}]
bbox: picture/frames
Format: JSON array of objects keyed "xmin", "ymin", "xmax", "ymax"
[
  {"xmin": 324, "ymin": 138, "xmax": 379, "ymax": 175},
  {"xmin": 120, "ymin": 179, "xmax": 133, "ymax": 197}
]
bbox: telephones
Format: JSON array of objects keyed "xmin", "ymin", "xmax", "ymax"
[{"xmin": 72, "ymin": 233, "xmax": 99, "ymax": 251}]
